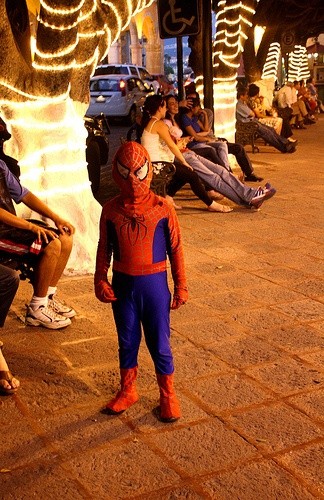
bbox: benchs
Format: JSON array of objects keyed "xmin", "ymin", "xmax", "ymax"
[
  {"xmin": 234, "ymin": 121, "xmax": 261, "ymax": 153},
  {"xmin": 149, "ymin": 160, "xmax": 176, "ymax": 198}
]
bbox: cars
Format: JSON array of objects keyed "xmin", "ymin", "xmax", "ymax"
[
  {"xmin": 86, "ymin": 76, "xmax": 154, "ymax": 124},
  {"xmin": 150, "ymin": 74, "xmax": 178, "ymax": 96}
]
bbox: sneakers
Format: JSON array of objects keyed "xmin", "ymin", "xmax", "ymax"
[
  {"xmin": 47, "ymin": 298, "xmax": 76, "ymax": 318},
  {"xmin": 250, "ymin": 183, "xmax": 276, "ymax": 209},
  {"xmin": 24, "ymin": 304, "xmax": 71, "ymax": 330}
]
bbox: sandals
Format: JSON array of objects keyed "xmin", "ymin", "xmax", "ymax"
[{"xmin": 0, "ymin": 370, "xmax": 20, "ymax": 393}]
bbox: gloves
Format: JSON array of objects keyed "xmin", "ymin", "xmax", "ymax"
[
  {"xmin": 94, "ymin": 281, "xmax": 117, "ymax": 304},
  {"xmin": 170, "ymin": 286, "xmax": 188, "ymax": 309}
]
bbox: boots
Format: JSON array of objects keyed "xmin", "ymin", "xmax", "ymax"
[
  {"xmin": 155, "ymin": 372, "xmax": 181, "ymax": 422},
  {"xmin": 106, "ymin": 366, "xmax": 139, "ymax": 414}
]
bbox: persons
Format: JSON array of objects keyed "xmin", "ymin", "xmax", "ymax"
[
  {"xmin": 237, "ymin": 82, "xmax": 298, "ymax": 154},
  {"xmin": 163, "ymin": 94, "xmax": 276, "ymax": 209},
  {"xmin": 277, "ymin": 81, "xmax": 296, "ymax": 142},
  {"xmin": 94, "ymin": 141, "xmax": 188, "ymax": 421},
  {"xmin": 140, "ymin": 94, "xmax": 233, "ymax": 212},
  {"xmin": 0, "ymin": 159, "xmax": 76, "ymax": 329},
  {"xmin": 180, "ymin": 94, "xmax": 263, "ymax": 182},
  {"xmin": 274, "ymin": 77, "xmax": 324, "ymax": 130}
]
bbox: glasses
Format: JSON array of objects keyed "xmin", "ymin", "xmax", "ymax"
[{"xmin": 170, "ymin": 101, "xmax": 178, "ymax": 105}]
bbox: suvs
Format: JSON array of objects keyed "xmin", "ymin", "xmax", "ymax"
[{"xmin": 95, "ymin": 64, "xmax": 162, "ymax": 96}]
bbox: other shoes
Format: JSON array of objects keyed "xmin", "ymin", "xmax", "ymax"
[
  {"xmin": 286, "ymin": 137, "xmax": 296, "ymax": 142},
  {"xmin": 283, "ymin": 140, "xmax": 298, "ymax": 152},
  {"xmin": 165, "ymin": 196, "xmax": 182, "ymax": 210},
  {"xmin": 289, "ymin": 115, "xmax": 316, "ymax": 129},
  {"xmin": 209, "ymin": 205, "xmax": 233, "ymax": 213},
  {"xmin": 208, "ymin": 191, "xmax": 224, "ymax": 200},
  {"xmin": 244, "ymin": 172, "xmax": 264, "ymax": 182}
]
